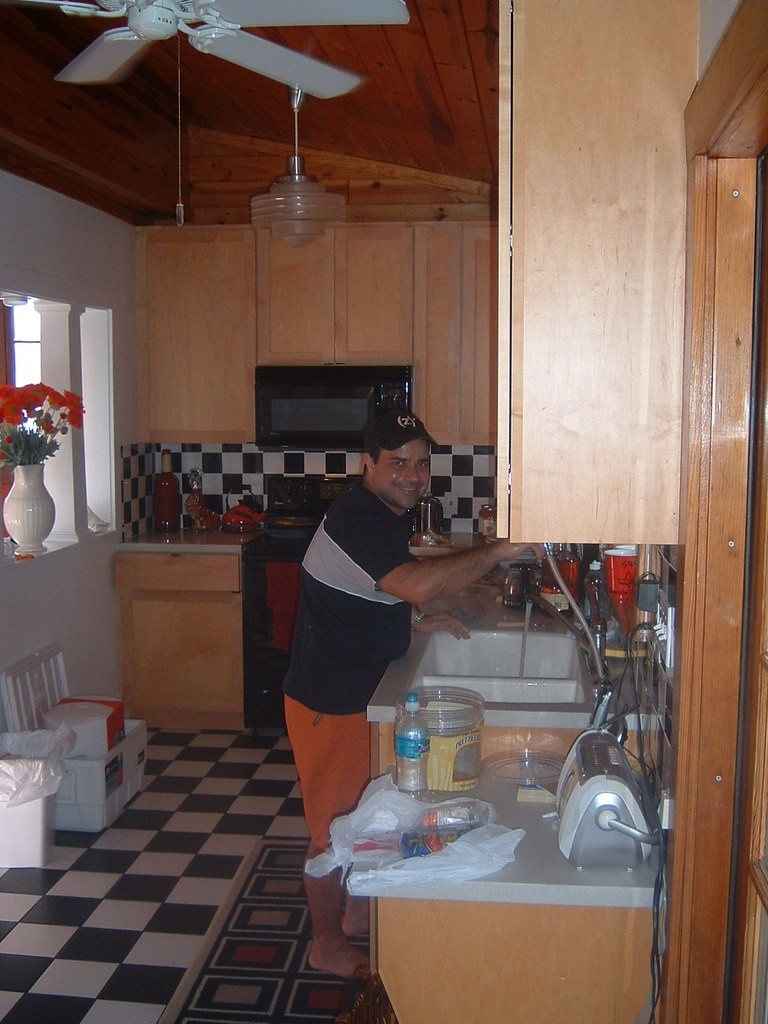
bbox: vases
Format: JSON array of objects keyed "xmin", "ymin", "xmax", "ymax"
[{"xmin": 2, "ymin": 464, "xmax": 56, "ymax": 553}]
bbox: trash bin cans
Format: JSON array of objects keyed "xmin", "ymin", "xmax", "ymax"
[{"xmin": 0, "ymin": 728, "xmax": 74, "ymax": 867}]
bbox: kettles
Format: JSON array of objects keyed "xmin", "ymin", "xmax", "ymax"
[{"xmin": 222, "ymin": 484, "xmax": 265, "ymax": 534}]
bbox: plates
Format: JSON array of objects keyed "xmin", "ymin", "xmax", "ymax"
[{"xmin": 498, "ymin": 560, "xmax": 542, "ymax": 569}]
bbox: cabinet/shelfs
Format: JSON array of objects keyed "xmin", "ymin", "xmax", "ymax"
[
  {"xmin": 411, "ymin": 220, "xmax": 498, "ymax": 446},
  {"xmin": 370, "ymin": 722, "xmax": 666, "ymax": 1024},
  {"xmin": 115, "ymin": 528, "xmax": 265, "ymax": 731},
  {"xmin": 255, "ymin": 222, "xmax": 413, "ymax": 366},
  {"xmin": 497, "ymin": 0, "xmax": 702, "ymax": 543},
  {"xmin": 133, "ymin": 224, "xmax": 272, "ymax": 444}
]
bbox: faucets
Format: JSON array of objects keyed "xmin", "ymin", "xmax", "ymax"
[{"xmin": 521, "ymin": 591, "xmax": 608, "ymax": 676}]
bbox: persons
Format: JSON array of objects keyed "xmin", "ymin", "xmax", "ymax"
[{"xmin": 282, "ymin": 410, "xmax": 545, "ymax": 983}]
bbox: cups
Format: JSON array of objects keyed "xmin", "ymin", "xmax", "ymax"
[
  {"xmin": 604, "ymin": 549, "xmax": 638, "ymax": 601},
  {"xmin": 615, "ymin": 545, "xmax": 637, "ymax": 549},
  {"xmin": 502, "ymin": 564, "xmax": 531, "ymax": 609}
]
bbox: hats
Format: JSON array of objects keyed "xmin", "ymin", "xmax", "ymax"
[{"xmin": 361, "ymin": 408, "xmax": 439, "ymax": 451}]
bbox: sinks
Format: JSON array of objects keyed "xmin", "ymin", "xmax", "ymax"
[
  {"xmin": 412, "ymin": 679, "xmax": 583, "ymax": 704},
  {"xmin": 414, "ymin": 630, "xmax": 577, "ymax": 679}
]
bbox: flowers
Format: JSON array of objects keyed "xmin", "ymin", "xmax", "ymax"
[{"xmin": 0, "ymin": 383, "xmax": 85, "ymax": 473}]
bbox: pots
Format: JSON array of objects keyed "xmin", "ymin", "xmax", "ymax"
[{"xmin": 234, "ymin": 511, "xmax": 319, "ymax": 540}]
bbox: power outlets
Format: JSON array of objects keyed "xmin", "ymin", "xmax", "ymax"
[
  {"xmin": 443, "ymin": 492, "xmax": 459, "ymax": 519},
  {"xmin": 654, "ymin": 589, "xmax": 674, "ymax": 667}
]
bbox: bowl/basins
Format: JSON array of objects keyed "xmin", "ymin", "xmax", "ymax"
[{"xmin": 416, "ymin": 492, "xmax": 442, "ymax": 534}]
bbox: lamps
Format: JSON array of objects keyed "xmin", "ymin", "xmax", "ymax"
[{"xmin": 248, "ymin": 88, "xmax": 347, "ymax": 248}]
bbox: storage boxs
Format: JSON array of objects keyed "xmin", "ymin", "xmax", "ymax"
[{"xmin": 0, "ymin": 718, "xmax": 147, "ymax": 833}]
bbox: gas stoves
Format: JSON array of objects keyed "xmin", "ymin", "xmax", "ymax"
[{"xmin": 263, "ymin": 474, "xmax": 365, "ymax": 561}]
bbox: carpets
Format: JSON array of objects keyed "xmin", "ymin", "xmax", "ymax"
[{"xmin": 155, "ymin": 838, "xmax": 370, "ymax": 1024}]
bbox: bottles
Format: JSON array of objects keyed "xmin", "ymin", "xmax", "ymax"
[
  {"xmin": 477, "ymin": 504, "xmax": 495, "ymax": 538},
  {"xmin": 155, "ymin": 449, "xmax": 180, "ymax": 533},
  {"xmin": 540, "ymin": 543, "xmax": 580, "ymax": 619},
  {"xmin": 414, "ymin": 686, "xmax": 485, "ymax": 794},
  {"xmin": 585, "ymin": 561, "xmax": 606, "ymax": 624},
  {"xmin": 395, "ymin": 693, "xmax": 430, "ymax": 799}
]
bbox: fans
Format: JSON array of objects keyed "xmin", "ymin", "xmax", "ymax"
[{"xmin": 15, "ymin": 0, "xmax": 410, "ymax": 227}]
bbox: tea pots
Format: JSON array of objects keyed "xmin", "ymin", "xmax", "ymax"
[{"xmin": 520, "ymin": 548, "xmax": 538, "ymax": 560}]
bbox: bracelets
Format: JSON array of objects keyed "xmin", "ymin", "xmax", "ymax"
[{"xmin": 411, "ymin": 610, "xmax": 426, "ymax": 630}]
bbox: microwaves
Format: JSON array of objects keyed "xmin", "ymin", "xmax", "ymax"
[{"xmin": 255, "ymin": 365, "xmax": 413, "ymax": 451}]
